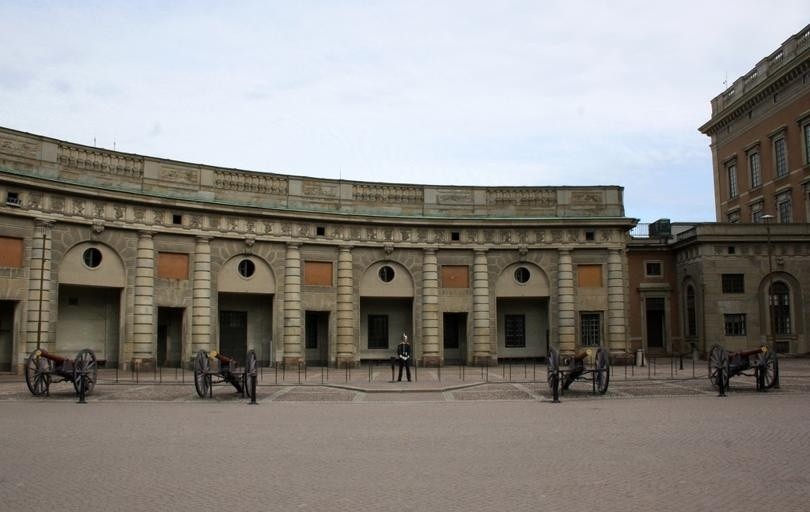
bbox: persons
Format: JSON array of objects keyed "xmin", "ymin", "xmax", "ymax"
[{"xmin": 397, "ymin": 336, "xmax": 411, "ymax": 382}]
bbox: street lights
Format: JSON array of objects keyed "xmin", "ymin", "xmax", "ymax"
[
  {"xmin": 757, "ymin": 213, "xmax": 783, "ymax": 388},
  {"xmin": 32, "ymin": 216, "xmax": 56, "ymax": 396}
]
